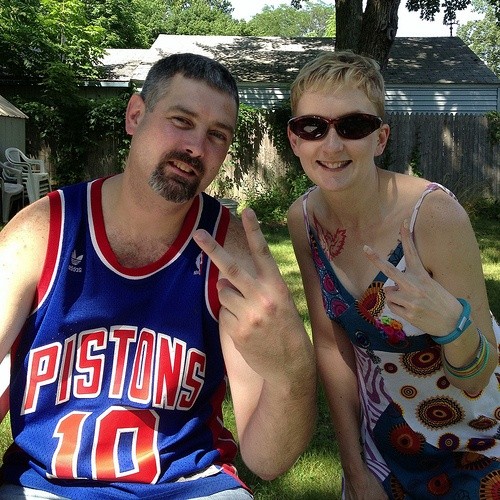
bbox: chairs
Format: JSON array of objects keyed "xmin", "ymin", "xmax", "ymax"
[{"xmin": 0, "ymin": 147, "xmax": 53, "ymax": 222}]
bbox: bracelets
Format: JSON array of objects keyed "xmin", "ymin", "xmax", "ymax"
[{"xmin": 434, "ymin": 298, "xmax": 490, "ymax": 381}]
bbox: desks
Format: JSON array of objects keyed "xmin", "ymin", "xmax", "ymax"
[{"xmin": 217, "ymin": 199, "xmax": 239, "ymax": 216}]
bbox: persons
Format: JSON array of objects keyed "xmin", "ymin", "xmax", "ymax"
[
  {"xmin": 286, "ymin": 50, "xmax": 500, "ymax": 500},
  {"xmin": 0, "ymin": 56, "xmax": 318, "ymax": 500}
]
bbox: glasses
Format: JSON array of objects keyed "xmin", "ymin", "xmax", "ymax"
[{"xmin": 288, "ymin": 113, "xmax": 383, "ymax": 141}]
